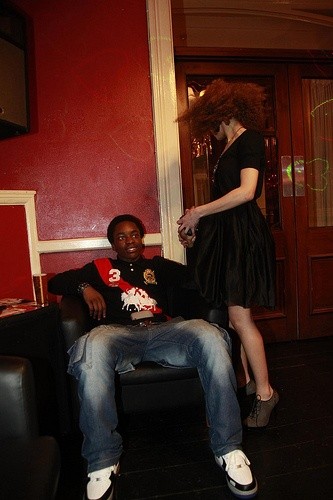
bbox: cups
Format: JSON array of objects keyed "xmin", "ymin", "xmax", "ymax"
[{"xmin": 33, "ymin": 274, "xmax": 48, "ymax": 305}]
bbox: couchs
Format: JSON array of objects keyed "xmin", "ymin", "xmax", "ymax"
[
  {"xmin": 59, "ymin": 293, "xmax": 235, "ymax": 426},
  {"xmin": 0, "ymin": 355, "xmax": 61, "ymax": 500}
]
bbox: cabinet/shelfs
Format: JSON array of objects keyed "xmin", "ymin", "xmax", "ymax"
[{"xmin": 0, "ymin": 1, "xmax": 29, "ymax": 139}]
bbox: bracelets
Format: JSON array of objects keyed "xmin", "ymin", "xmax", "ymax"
[{"xmin": 77, "ymin": 282, "xmax": 90, "ymax": 294}]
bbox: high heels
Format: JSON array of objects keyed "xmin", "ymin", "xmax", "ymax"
[
  {"xmin": 245, "ymin": 379, "xmax": 257, "ymax": 401},
  {"xmin": 242, "ymin": 389, "xmax": 279, "ymax": 429}
]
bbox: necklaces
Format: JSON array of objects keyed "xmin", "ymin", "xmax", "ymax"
[{"xmin": 212, "ymin": 127, "xmax": 244, "ymax": 183}]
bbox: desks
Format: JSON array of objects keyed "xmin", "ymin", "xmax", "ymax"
[{"xmin": 0, "ymin": 301, "xmax": 60, "ymax": 410}]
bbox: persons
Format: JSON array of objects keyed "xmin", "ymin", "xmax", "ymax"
[
  {"xmin": 47, "ymin": 214, "xmax": 258, "ymax": 500},
  {"xmin": 177, "ymin": 79, "xmax": 281, "ymax": 431}
]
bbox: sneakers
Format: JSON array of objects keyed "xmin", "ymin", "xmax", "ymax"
[
  {"xmin": 215, "ymin": 449, "xmax": 258, "ymax": 500},
  {"xmin": 83, "ymin": 462, "xmax": 121, "ymax": 500}
]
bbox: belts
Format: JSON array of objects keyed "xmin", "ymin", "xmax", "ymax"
[{"xmin": 133, "ymin": 320, "xmax": 162, "ymax": 326}]
